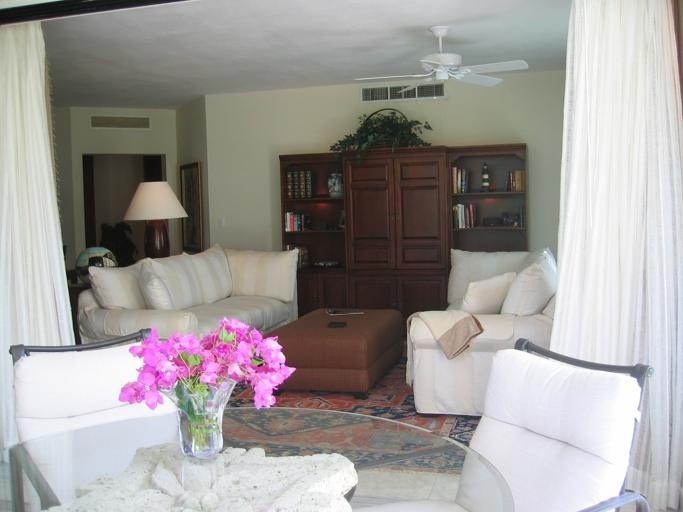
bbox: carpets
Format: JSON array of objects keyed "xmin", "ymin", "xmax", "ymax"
[{"xmin": 224, "ymin": 357, "xmax": 480, "ymax": 475}]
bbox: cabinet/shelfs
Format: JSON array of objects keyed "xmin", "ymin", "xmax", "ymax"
[
  {"xmin": 278, "ymin": 152, "xmax": 347, "ymax": 272},
  {"xmin": 446, "ymin": 143, "xmax": 531, "ymax": 251},
  {"xmin": 296, "ymin": 271, "xmax": 349, "ymax": 314},
  {"xmin": 349, "ymin": 271, "xmax": 446, "ymax": 337},
  {"xmin": 344, "ymin": 146, "xmax": 447, "ymax": 271}
]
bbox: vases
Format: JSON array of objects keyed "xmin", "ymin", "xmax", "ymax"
[
  {"xmin": 327, "ymin": 168, "xmax": 343, "ymax": 198},
  {"xmin": 159, "ymin": 381, "xmax": 237, "ymax": 458}
]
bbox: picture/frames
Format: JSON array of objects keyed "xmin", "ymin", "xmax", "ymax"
[{"xmin": 177, "ymin": 161, "xmax": 203, "ymax": 254}]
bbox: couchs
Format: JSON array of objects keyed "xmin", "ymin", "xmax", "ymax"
[
  {"xmin": 75, "ymin": 243, "xmax": 301, "ymax": 346},
  {"xmin": 400, "ymin": 274, "xmax": 557, "ymax": 415}
]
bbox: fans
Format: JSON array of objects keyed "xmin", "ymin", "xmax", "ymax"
[{"xmin": 353, "ymin": 24, "xmax": 530, "ymax": 99}]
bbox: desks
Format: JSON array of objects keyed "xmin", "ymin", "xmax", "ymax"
[{"xmin": 6, "ymin": 408, "xmax": 517, "ymax": 512}]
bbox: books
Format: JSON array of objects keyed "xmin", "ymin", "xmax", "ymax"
[
  {"xmin": 507, "ymin": 169, "xmax": 525, "ymax": 192},
  {"xmin": 451, "ymin": 167, "xmax": 477, "ymax": 229},
  {"xmin": 285, "ymin": 170, "xmax": 312, "ymax": 269}
]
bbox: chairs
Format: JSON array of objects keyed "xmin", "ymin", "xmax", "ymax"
[
  {"xmin": 353, "ymin": 337, "xmax": 657, "ymax": 512},
  {"xmin": 10, "ymin": 327, "xmax": 178, "ymax": 511}
]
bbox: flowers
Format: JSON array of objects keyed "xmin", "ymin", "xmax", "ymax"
[{"xmin": 115, "ymin": 315, "xmax": 296, "ymax": 445}]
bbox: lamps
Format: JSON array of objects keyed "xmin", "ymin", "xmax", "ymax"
[{"xmin": 121, "ymin": 179, "xmax": 190, "ymax": 260}]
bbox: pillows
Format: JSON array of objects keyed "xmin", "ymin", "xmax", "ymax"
[
  {"xmin": 192, "ymin": 244, "xmax": 232, "ymax": 305},
  {"xmin": 445, "ymin": 246, "xmax": 529, "ymax": 306},
  {"xmin": 139, "ymin": 263, "xmax": 177, "ymax": 311},
  {"xmin": 462, "ymin": 272, "xmax": 516, "ymax": 317},
  {"xmin": 501, "ymin": 245, "xmax": 558, "ymax": 317},
  {"xmin": 155, "ymin": 253, "xmax": 202, "ymax": 311},
  {"xmin": 84, "ymin": 259, "xmax": 156, "ymax": 313},
  {"xmin": 225, "ymin": 249, "xmax": 298, "ymax": 304}
]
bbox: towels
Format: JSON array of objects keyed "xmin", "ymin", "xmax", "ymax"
[{"xmin": 405, "ymin": 309, "xmax": 482, "ymax": 387}]
